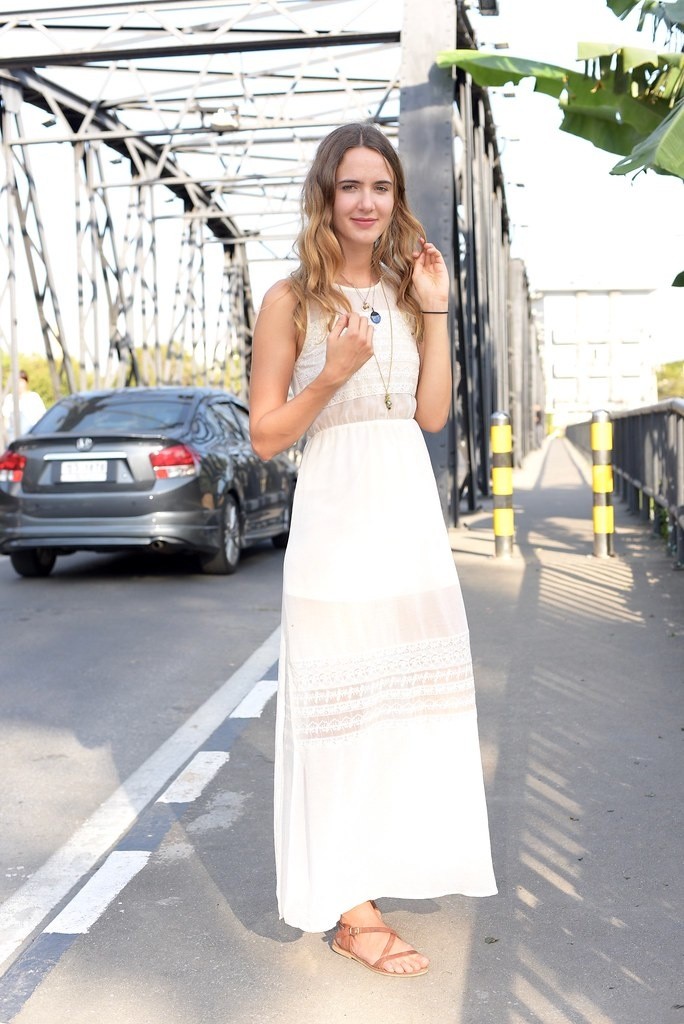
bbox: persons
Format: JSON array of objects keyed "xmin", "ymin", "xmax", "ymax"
[{"xmin": 247, "ymin": 124, "xmax": 497, "ymax": 975}]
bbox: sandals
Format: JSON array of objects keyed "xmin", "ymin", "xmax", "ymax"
[
  {"xmin": 331, "ymin": 920, "xmax": 428, "ymax": 977},
  {"xmin": 371, "ymin": 901, "xmax": 382, "ymax": 921}
]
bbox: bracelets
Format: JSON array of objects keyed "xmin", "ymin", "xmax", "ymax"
[{"xmin": 420, "ymin": 311, "xmax": 450, "ymax": 315}]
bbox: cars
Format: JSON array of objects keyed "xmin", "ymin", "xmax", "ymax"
[{"xmin": 0, "ymin": 387, "xmax": 299, "ymax": 575}]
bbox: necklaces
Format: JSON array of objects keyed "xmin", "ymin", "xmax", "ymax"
[{"xmin": 334, "ymin": 265, "xmax": 393, "ymax": 410}]
